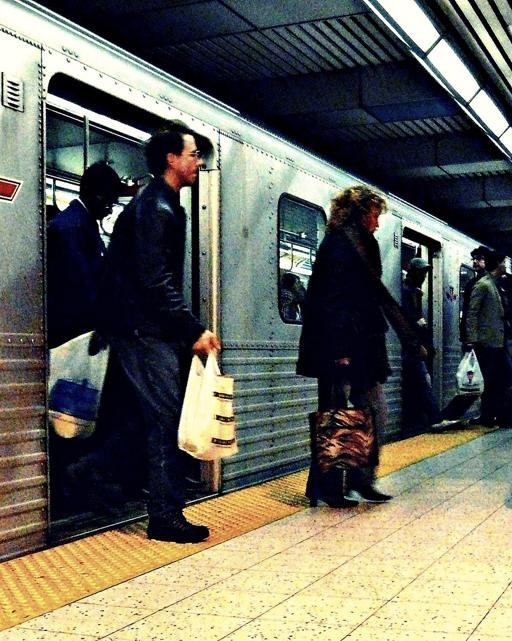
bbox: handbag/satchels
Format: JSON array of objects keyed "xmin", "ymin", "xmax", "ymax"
[{"xmin": 309, "ymin": 408, "xmax": 378, "ymax": 473}]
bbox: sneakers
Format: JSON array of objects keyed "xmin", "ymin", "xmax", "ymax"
[{"xmin": 147, "ymin": 511, "xmax": 209, "ymax": 542}]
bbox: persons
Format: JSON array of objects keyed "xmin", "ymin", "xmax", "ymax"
[
  {"xmin": 74, "ymin": 130, "xmax": 221, "ymax": 543},
  {"xmin": 279, "ymin": 273, "xmax": 306, "ymax": 321},
  {"xmin": 295, "ymin": 186, "xmax": 428, "ymax": 508},
  {"xmin": 402, "ymin": 245, "xmax": 512, "ymax": 429},
  {"xmin": 47, "ymin": 161, "xmax": 126, "ymax": 505}
]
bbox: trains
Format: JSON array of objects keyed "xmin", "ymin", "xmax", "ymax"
[{"xmin": 0, "ymin": 0, "xmax": 511, "ymax": 562}]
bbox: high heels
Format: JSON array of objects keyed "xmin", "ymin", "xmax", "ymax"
[
  {"xmin": 344, "ymin": 482, "xmax": 392, "ymax": 501},
  {"xmin": 308, "ymin": 494, "xmax": 359, "ymax": 508}
]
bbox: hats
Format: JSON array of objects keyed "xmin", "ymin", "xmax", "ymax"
[{"xmin": 409, "ymin": 258, "xmax": 431, "ymax": 268}]
